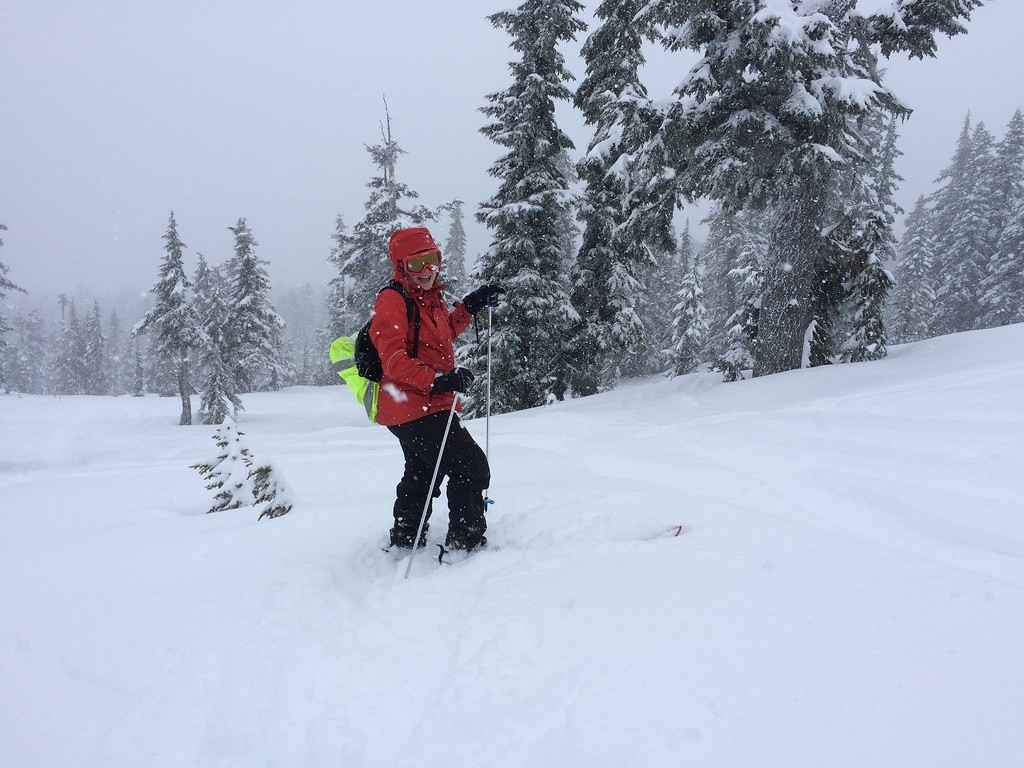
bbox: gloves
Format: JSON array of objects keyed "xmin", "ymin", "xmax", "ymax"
[
  {"xmin": 432, "ymin": 367, "xmax": 475, "ymax": 393},
  {"xmin": 462, "ymin": 284, "xmax": 507, "ymax": 315}
]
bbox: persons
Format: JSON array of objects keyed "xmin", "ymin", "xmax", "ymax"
[{"xmin": 355, "ymin": 228, "xmax": 509, "ymax": 562}]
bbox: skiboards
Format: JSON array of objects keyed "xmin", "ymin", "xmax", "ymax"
[{"xmin": 381, "ymin": 522, "xmax": 680, "ymax": 568}]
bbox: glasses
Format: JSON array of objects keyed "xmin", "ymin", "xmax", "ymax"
[{"xmin": 398, "ymin": 249, "xmax": 442, "ymax": 276}]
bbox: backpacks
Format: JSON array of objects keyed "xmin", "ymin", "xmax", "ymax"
[{"xmin": 353, "ymin": 279, "xmax": 420, "ymax": 383}]
